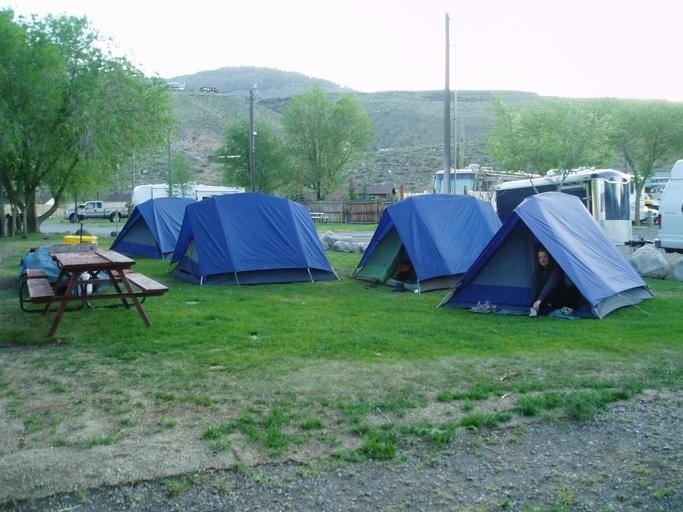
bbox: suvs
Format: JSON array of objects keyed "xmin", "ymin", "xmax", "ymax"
[
  {"xmin": 654, "ymin": 158, "xmax": 682, "ymax": 251},
  {"xmin": 166, "ymin": 82, "xmax": 184, "ymax": 90}
]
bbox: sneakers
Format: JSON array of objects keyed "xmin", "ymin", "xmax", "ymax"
[{"xmin": 471, "ymin": 301, "xmax": 497, "ymax": 314}]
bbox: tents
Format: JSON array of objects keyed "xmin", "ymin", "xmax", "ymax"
[
  {"xmin": 170, "ymin": 190, "xmax": 340, "ymax": 287},
  {"xmin": 349, "ymin": 193, "xmax": 504, "ymax": 295},
  {"xmin": 108, "ymin": 195, "xmax": 200, "ymax": 261},
  {"xmin": 438, "ymin": 190, "xmax": 658, "ymax": 321}
]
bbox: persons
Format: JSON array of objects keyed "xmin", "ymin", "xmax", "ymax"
[{"xmin": 528, "ymin": 244, "xmax": 566, "ymax": 319}]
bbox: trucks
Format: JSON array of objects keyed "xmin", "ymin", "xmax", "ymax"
[{"xmin": 131, "ymin": 183, "xmax": 244, "ymax": 209}]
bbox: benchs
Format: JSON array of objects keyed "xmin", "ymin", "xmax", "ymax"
[
  {"xmin": 110, "ymin": 270, "xmax": 132, "ymax": 279},
  {"xmin": 19, "ymin": 268, "xmax": 47, "ymax": 279},
  {"xmin": 19, "ymin": 279, "xmax": 54, "ymax": 313},
  {"xmin": 127, "ymin": 272, "xmax": 169, "ymax": 306}
]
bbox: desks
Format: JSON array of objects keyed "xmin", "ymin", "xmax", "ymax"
[{"xmin": 42, "ymin": 250, "xmax": 153, "ymax": 338}]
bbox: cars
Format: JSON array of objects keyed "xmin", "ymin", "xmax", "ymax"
[
  {"xmin": 199, "ymin": 87, "xmax": 217, "ymax": 93},
  {"xmin": 310, "ymin": 212, "xmax": 328, "ymax": 223},
  {"xmin": 643, "ymin": 177, "xmax": 668, "ymax": 223}
]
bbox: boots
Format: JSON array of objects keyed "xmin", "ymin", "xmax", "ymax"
[{"xmin": 392, "ymin": 281, "xmax": 404, "ymax": 292}]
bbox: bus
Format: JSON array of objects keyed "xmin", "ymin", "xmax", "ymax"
[{"xmin": 431, "ymin": 166, "xmax": 542, "ymax": 200}]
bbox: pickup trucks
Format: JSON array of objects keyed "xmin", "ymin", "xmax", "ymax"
[{"xmin": 63, "ymin": 200, "xmax": 128, "ymax": 224}]
